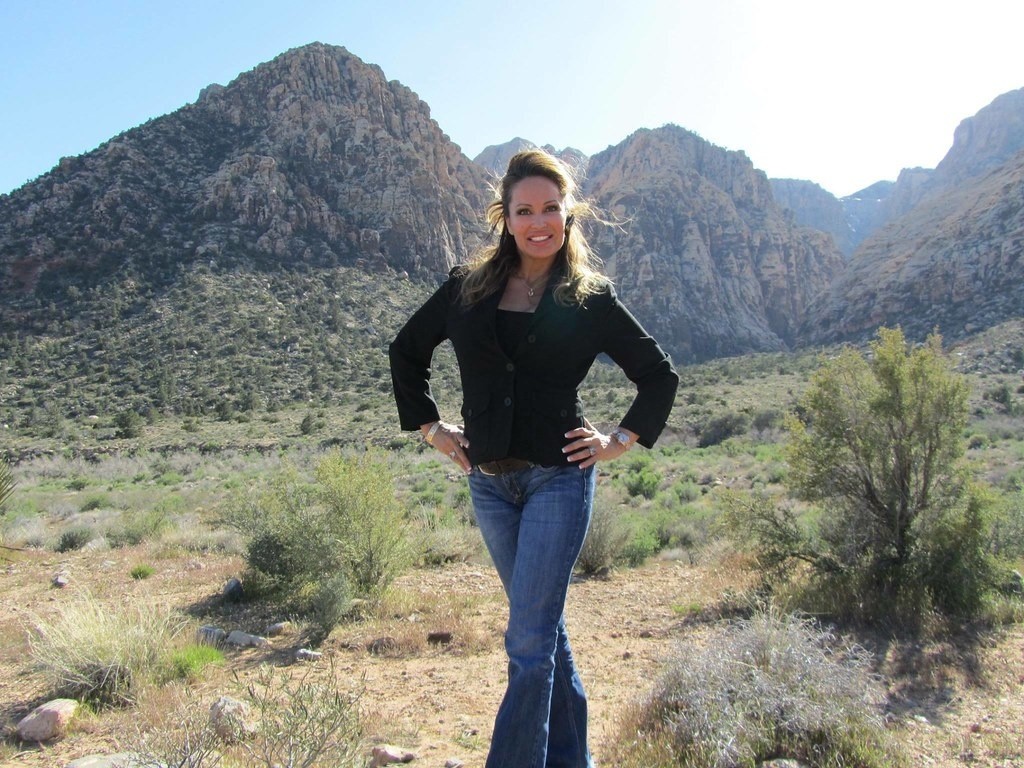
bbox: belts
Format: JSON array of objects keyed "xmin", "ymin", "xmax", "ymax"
[{"xmin": 477, "ymin": 457, "xmax": 533, "ymax": 475}]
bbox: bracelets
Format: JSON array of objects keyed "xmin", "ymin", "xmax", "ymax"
[{"xmin": 426, "ymin": 421, "xmax": 444, "ymax": 442}]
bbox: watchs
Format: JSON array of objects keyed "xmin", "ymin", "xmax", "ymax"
[{"xmin": 612, "ymin": 429, "xmax": 633, "ymax": 451}]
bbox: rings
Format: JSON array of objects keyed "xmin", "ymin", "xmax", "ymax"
[
  {"xmin": 449, "ymin": 450, "xmax": 457, "ymax": 459},
  {"xmin": 588, "ymin": 447, "xmax": 596, "ymax": 456}
]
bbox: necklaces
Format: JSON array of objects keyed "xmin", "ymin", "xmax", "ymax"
[{"xmin": 519, "ymin": 274, "xmax": 546, "ymax": 295}]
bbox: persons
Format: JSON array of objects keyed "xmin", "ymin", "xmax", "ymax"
[{"xmin": 388, "ymin": 152, "xmax": 679, "ymax": 768}]
